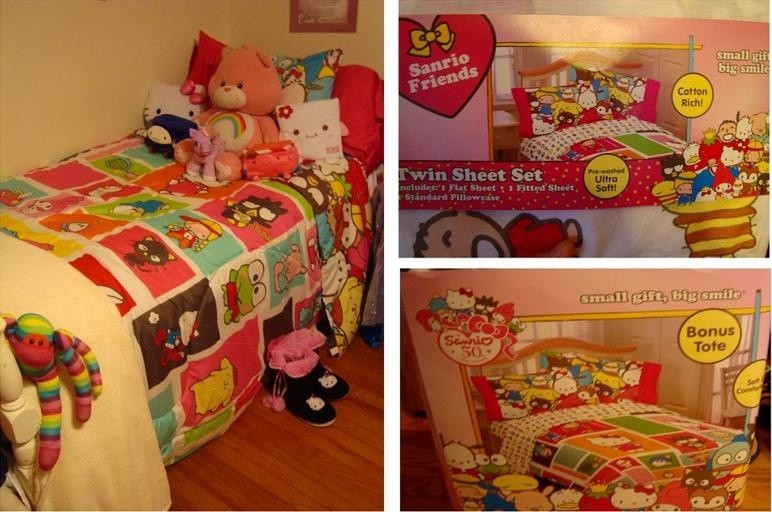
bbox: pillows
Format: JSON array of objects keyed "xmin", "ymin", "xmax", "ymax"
[
  {"xmin": 470, "ymin": 352, "xmax": 662, "ymax": 422},
  {"xmin": 511, "ymin": 70, "xmax": 661, "ymax": 140},
  {"xmin": 181, "ymin": 30, "xmax": 383, "ymax": 160}
]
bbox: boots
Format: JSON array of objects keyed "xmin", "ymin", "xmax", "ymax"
[
  {"xmin": 284, "ymin": 328, "xmax": 350, "ymax": 400},
  {"xmin": 263, "ymin": 344, "xmax": 336, "ymax": 427}
]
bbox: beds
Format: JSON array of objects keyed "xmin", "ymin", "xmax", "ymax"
[
  {"xmin": 514, "ymin": 51, "xmax": 686, "ymax": 160},
  {"xmin": 471, "ymin": 337, "xmax": 751, "ymax": 494},
  {"xmin": 0, "ymin": 118, "xmax": 384, "ymax": 512}
]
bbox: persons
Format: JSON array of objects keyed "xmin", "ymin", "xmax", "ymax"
[{"xmin": 136, "ymin": 42, "xmax": 299, "ymax": 182}]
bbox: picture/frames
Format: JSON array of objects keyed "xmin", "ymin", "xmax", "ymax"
[{"xmin": 290, "ymin": 0, "xmax": 358, "ymax": 32}]
